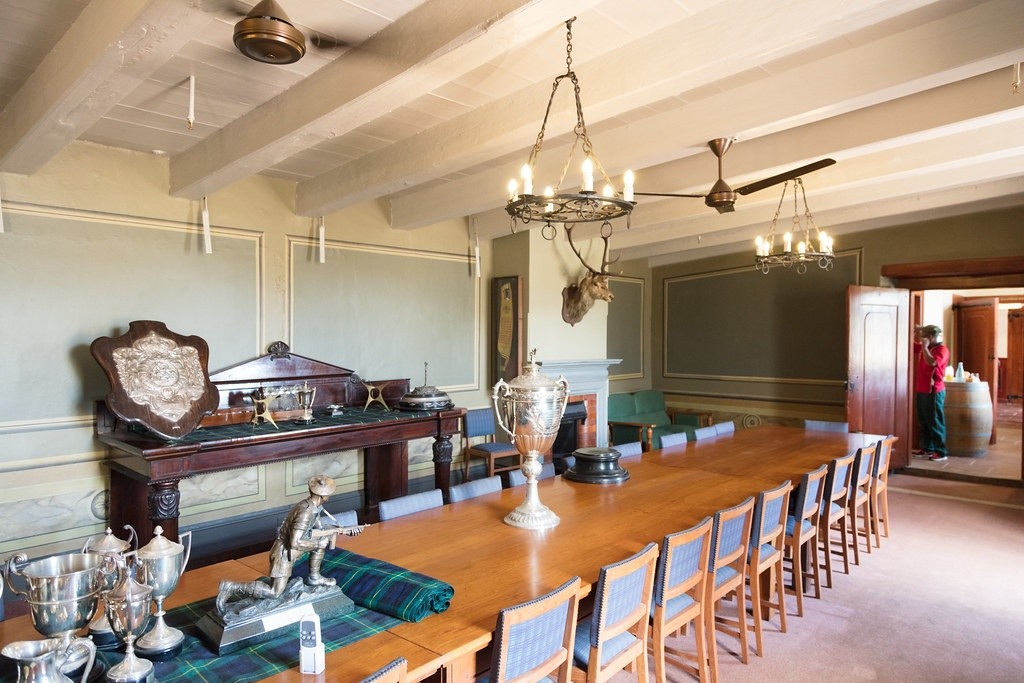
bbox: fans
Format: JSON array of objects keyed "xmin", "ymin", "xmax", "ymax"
[
  {"xmin": 180, "ymin": 0, "xmax": 428, "ymax": 64},
  {"xmin": 615, "ymin": 139, "xmax": 837, "ymax": 214}
]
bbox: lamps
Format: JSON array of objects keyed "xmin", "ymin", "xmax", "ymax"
[
  {"xmin": 753, "ymin": 176, "xmax": 835, "ymax": 272},
  {"xmin": 504, "ymin": 14, "xmax": 637, "ymax": 242}
]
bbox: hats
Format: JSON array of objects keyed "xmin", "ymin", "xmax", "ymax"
[{"xmin": 919, "ymin": 325, "xmax": 942, "ymax": 340}]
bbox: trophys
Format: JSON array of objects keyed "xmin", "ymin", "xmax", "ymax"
[
  {"xmin": 1, "ymin": 523, "xmax": 193, "ymax": 683},
  {"xmin": 492, "ymin": 349, "xmax": 572, "ymax": 530}
]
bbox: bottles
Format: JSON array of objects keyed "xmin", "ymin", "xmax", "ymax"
[{"xmin": 956, "ymin": 362, "xmax": 965, "ymax": 383}]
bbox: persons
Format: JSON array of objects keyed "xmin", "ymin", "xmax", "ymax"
[
  {"xmin": 913, "ymin": 324, "xmax": 951, "ymax": 463},
  {"xmin": 215, "ymin": 476, "xmax": 365, "ymax": 616}
]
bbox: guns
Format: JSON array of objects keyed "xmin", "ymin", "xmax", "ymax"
[{"xmin": 308, "ymin": 524, "xmax": 371, "ymax": 551}]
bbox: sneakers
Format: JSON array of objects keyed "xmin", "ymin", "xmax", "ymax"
[
  {"xmin": 929, "ymin": 452, "xmax": 948, "ymax": 462},
  {"xmin": 911, "ymin": 449, "xmax": 934, "ymax": 458}
]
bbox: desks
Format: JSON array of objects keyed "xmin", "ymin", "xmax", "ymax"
[
  {"xmin": 93, "ymin": 341, "xmax": 468, "ymax": 556},
  {"xmin": 941, "ymin": 376, "xmax": 994, "ymax": 457}
]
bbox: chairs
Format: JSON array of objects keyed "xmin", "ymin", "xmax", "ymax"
[
  {"xmin": 1, "ymin": 419, "xmax": 898, "ymax": 683},
  {"xmin": 461, "ymin": 407, "xmax": 523, "ymax": 477}
]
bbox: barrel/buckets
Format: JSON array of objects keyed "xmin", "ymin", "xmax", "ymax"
[{"xmin": 942, "ymin": 382, "xmax": 994, "ymax": 456}]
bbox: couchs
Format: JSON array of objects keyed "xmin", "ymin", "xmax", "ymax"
[{"xmin": 608, "ymin": 388, "xmax": 714, "ymax": 451}]
